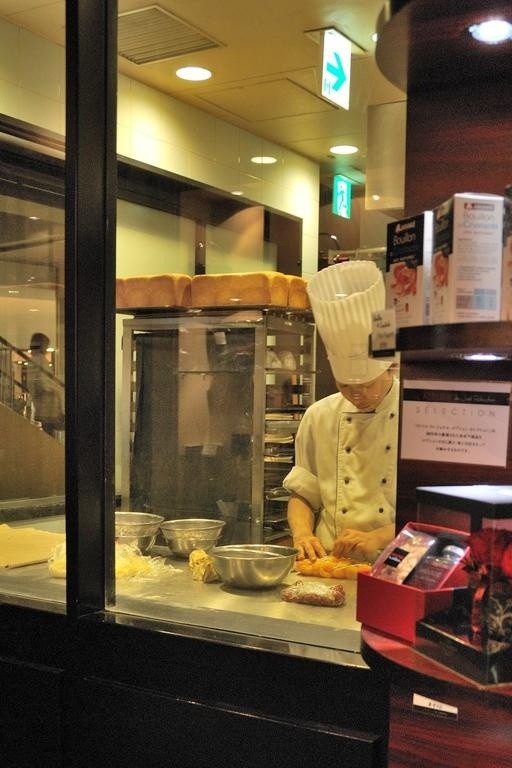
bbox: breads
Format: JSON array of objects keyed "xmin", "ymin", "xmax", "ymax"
[
  {"xmin": 189, "ymin": 547, "xmax": 221, "ymax": 583},
  {"xmin": 114, "ymin": 271, "xmax": 311, "ymax": 313},
  {"xmin": 280, "ymin": 580, "xmax": 345, "ymax": 607}
]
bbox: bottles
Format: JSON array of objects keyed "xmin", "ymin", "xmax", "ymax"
[{"xmin": 405, "ymin": 545, "xmax": 464, "ymax": 590}]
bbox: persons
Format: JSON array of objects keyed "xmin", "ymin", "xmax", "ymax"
[
  {"xmin": 284, "ymin": 356, "xmax": 402, "ymax": 565},
  {"xmin": 25, "ymin": 331, "xmax": 60, "ymax": 438}
]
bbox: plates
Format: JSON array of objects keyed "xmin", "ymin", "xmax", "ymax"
[{"xmin": 262, "ymin": 412, "xmax": 295, "ymax": 463}]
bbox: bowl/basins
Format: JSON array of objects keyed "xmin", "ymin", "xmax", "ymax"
[
  {"xmin": 165, "ymin": 538, "xmax": 219, "ymax": 557},
  {"xmin": 163, "ymin": 518, "xmax": 225, "ymax": 538},
  {"xmin": 209, "ymin": 543, "xmax": 299, "ymax": 589},
  {"xmin": 115, "ymin": 534, "xmax": 155, "ymax": 554},
  {"xmin": 113, "ymin": 509, "xmax": 164, "ymax": 534}
]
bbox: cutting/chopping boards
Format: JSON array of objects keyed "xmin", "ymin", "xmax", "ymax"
[{"xmin": 0, "ymin": 525, "xmax": 67, "ymax": 569}]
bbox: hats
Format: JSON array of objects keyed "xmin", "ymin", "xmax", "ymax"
[{"xmin": 306, "ymin": 260, "xmax": 395, "ymax": 385}]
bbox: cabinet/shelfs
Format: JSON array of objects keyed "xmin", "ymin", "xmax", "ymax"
[{"xmin": 360, "ymin": 0, "xmax": 511, "ymax": 767}]
